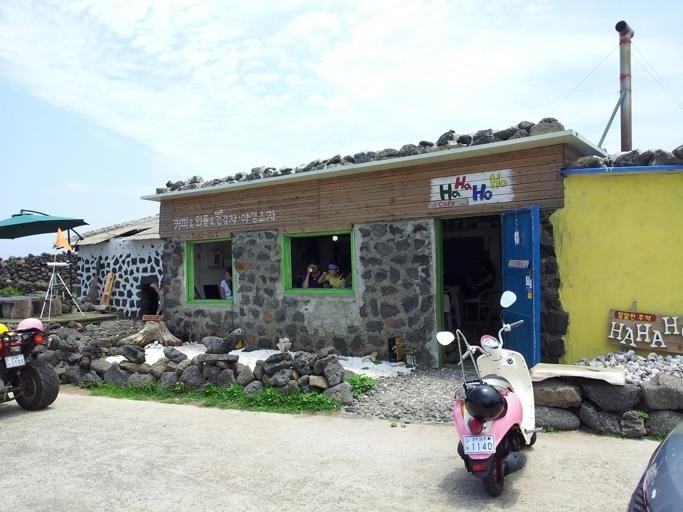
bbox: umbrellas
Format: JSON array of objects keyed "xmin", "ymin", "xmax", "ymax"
[{"xmin": 0, "ymin": 209, "xmax": 91, "ymax": 313}]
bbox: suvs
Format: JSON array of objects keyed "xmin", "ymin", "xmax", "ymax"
[{"xmin": 0, "ymin": 328, "xmax": 60, "ymax": 410}]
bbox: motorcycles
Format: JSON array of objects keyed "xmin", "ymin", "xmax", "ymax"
[{"xmin": 436, "ymin": 291, "xmax": 543, "ymax": 497}]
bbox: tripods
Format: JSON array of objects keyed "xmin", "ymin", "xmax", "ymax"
[{"xmin": 38, "ymin": 268, "xmax": 85, "ymax": 320}]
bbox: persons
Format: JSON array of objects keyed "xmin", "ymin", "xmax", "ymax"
[
  {"xmin": 292, "ymin": 259, "xmax": 352, "ymax": 289},
  {"xmin": 218, "ymin": 269, "xmax": 233, "ymax": 299}
]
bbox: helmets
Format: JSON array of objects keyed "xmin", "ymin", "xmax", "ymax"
[
  {"xmin": 17, "ymin": 318, "xmax": 43, "ymax": 331},
  {"xmin": 465, "ymin": 384, "xmax": 507, "ymax": 420}
]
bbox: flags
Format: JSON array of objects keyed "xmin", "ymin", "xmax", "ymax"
[{"xmin": 55, "ymin": 228, "xmax": 73, "ymax": 254}]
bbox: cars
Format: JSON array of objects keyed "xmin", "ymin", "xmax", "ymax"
[{"xmin": 626, "ymin": 421, "xmax": 683, "ymax": 512}]
